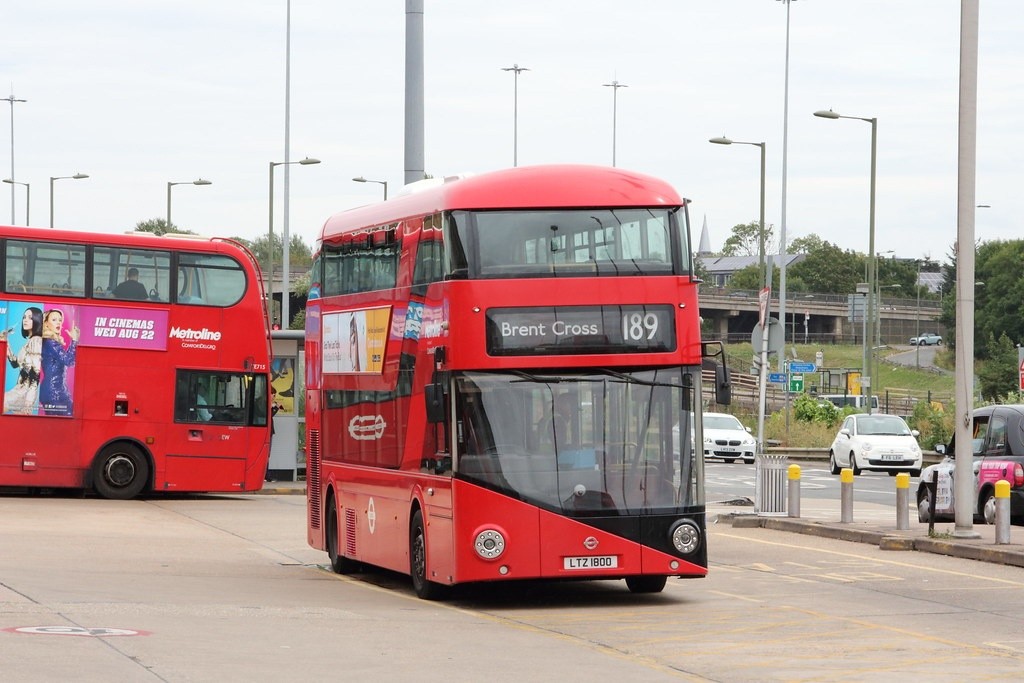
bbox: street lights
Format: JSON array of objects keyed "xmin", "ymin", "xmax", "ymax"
[
  {"xmin": 772, "ymin": 0, "xmax": 799, "ymax": 372},
  {"xmin": 812, "ymin": 108, "xmax": 879, "ymax": 412},
  {"xmin": 914, "ymin": 258, "xmax": 940, "ymax": 371},
  {"xmin": 2, "ymin": 178, "xmax": 32, "ymax": 227},
  {"xmin": 350, "ymin": 175, "xmax": 388, "ymax": 200},
  {"xmin": 792, "ymin": 294, "xmax": 814, "ymax": 360},
  {"xmin": 166, "ymin": 177, "xmax": 213, "ymax": 233},
  {"xmin": 501, "ymin": 63, "xmax": 533, "ymax": 166},
  {"xmin": 266, "ymin": 156, "xmax": 323, "ymax": 330},
  {"xmin": 600, "ymin": 80, "xmax": 629, "ymax": 166},
  {"xmin": 875, "ymin": 284, "xmax": 902, "ymax": 392},
  {"xmin": 875, "ymin": 248, "xmax": 896, "ymax": 347},
  {"xmin": 0, "ymin": 96, "xmax": 28, "ymax": 226},
  {"xmin": 49, "ymin": 172, "xmax": 91, "ymax": 228},
  {"xmin": 708, "ymin": 136, "xmax": 766, "ymax": 292}
]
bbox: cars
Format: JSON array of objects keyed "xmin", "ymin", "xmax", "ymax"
[
  {"xmin": 909, "ymin": 331, "xmax": 943, "ymax": 347},
  {"xmin": 828, "ymin": 413, "xmax": 924, "ymax": 477},
  {"xmin": 663, "ymin": 411, "xmax": 757, "ymax": 465},
  {"xmin": 914, "ymin": 404, "xmax": 1024, "ymax": 526}
]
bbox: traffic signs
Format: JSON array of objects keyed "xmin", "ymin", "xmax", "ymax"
[
  {"xmin": 767, "ymin": 373, "xmax": 787, "ymax": 383},
  {"xmin": 789, "ymin": 372, "xmax": 804, "ymax": 394},
  {"xmin": 789, "ymin": 362, "xmax": 817, "ymax": 373}
]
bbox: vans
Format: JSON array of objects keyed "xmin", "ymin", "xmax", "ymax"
[{"xmin": 813, "ymin": 394, "xmax": 879, "ymax": 414}]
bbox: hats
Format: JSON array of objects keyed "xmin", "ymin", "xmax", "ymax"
[{"xmin": 557, "ymin": 392, "xmax": 583, "ymax": 412}]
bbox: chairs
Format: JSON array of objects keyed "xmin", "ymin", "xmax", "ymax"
[
  {"xmin": 470, "ymin": 407, "xmax": 525, "ymax": 455},
  {"xmin": 8, "ymin": 280, "xmax": 162, "ymax": 301}
]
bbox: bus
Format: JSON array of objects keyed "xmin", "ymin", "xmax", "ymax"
[
  {"xmin": 0, "ymin": 225, "xmax": 275, "ymax": 502},
  {"xmin": 304, "ymin": 162, "xmax": 732, "ymax": 600}
]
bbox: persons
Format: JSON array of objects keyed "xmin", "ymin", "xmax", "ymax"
[
  {"xmin": 535, "ymin": 392, "xmax": 576, "ymax": 453},
  {"xmin": 0, "ymin": 306, "xmax": 43, "ymax": 415},
  {"xmin": 810, "ymin": 381, "xmax": 817, "ymax": 398},
  {"xmin": 109, "ymin": 267, "xmax": 153, "ymax": 301},
  {"xmin": 258, "ymin": 387, "xmax": 279, "ymax": 482},
  {"xmin": 39, "ymin": 309, "xmax": 80, "ymax": 417},
  {"xmin": 190, "ymin": 382, "xmax": 215, "ymax": 422}
]
